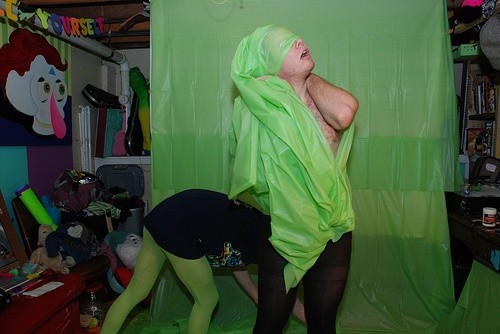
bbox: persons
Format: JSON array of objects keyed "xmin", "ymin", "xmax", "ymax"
[
  {"xmin": 99, "ymin": 189, "xmax": 306, "ymax": 334},
  {"xmin": 229, "ymin": 24, "xmax": 359, "ymax": 334}
]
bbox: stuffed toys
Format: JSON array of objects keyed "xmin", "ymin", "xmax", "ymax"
[
  {"xmin": 30, "ymin": 224, "xmax": 94, "ymax": 274},
  {"xmin": 115, "ymin": 232, "xmax": 143, "ymax": 269}
]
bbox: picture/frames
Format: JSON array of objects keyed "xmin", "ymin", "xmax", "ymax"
[{"xmin": 0, "ymin": 189, "xmax": 29, "ymax": 274}]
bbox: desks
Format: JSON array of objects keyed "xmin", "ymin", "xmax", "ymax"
[
  {"xmin": 0, "ymin": 265, "xmax": 98, "ymax": 334},
  {"xmin": 449, "ymin": 213, "xmax": 500, "ymax": 273}
]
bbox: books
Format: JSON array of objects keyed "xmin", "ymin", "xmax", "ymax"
[
  {"xmin": 465, "ymin": 115, "xmax": 500, "ymax": 158},
  {"xmin": 463, "ymin": 71, "xmax": 500, "ymax": 115}
]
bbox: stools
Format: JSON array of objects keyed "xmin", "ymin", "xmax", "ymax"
[{"xmin": 85, "ymin": 282, "xmax": 103, "ymax": 316}]
